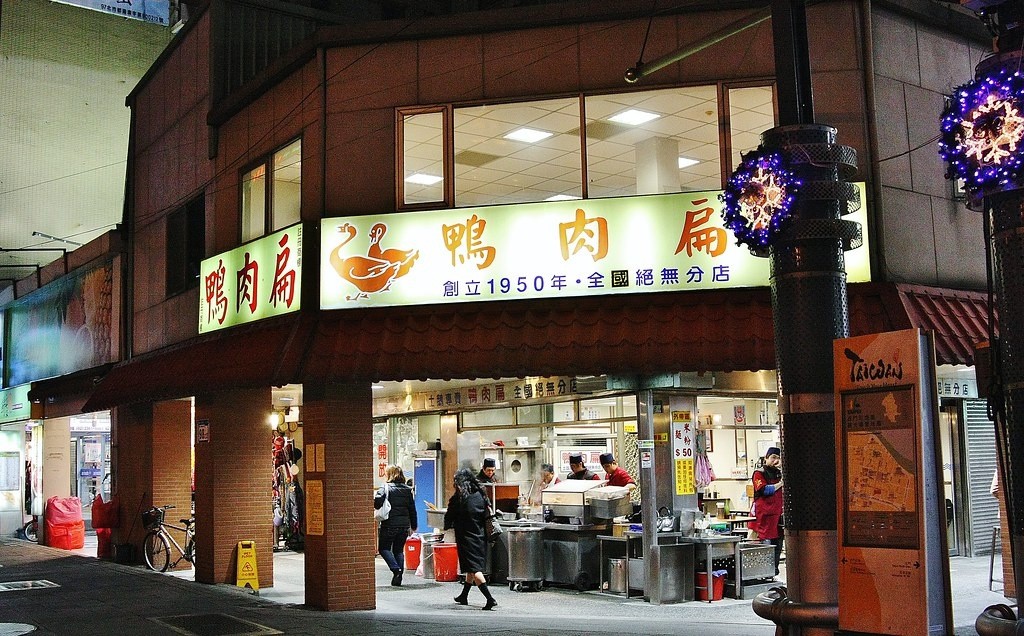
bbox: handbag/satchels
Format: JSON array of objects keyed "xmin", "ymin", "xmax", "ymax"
[
  {"xmin": 373, "ymin": 482, "xmax": 391, "ymax": 521},
  {"xmin": 483, "ymin": 505, "xmax": 503, "ymax": 541}
]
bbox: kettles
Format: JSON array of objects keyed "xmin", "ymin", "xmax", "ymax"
[{"xmin": 656, "ymin": 506, "xmax": 676, "ymax": 533}]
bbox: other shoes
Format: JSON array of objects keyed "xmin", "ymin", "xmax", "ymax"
[
  {"xmin": 482, "ymin": 597, "xmax": 497, "ymax": 609},
  {"xmin": 391, "ymin": 566, "xmax": 404, "ymax": 585},
  {"xmin": 454, "ymin": 595, "xmax": 468, "ymax": 604}
]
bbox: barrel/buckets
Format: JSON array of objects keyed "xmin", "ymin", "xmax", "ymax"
[
  {"xmin": 430, "ymin": 543, "xmax": 458, "ymax": 582},
  {"xmin": 607, "ymin": 557, "xmax": 626, "ymax": 593},
  {"xmin": 697, "ymin": 570, "xmax": 724, "ymax": 600},
  {"xmin": 405, "ymin": 539, "xmax": 421, "ymax": 570}
]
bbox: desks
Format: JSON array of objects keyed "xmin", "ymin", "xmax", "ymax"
[{"xmin": 712, "ymin": 515, "xmax": 756, "ymax": 535}]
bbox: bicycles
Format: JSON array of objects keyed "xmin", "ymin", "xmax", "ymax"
[
  {"xmin": 139, "ymin": 505, "xmax": 196, "ymax": 572},
  {"xmin": 23, "ymin": 517, "xmax": 39, "ymax": 543}
]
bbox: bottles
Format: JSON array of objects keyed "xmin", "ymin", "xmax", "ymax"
[
  {"xmin": 715, "ymin": 502, "xmax": 724, "ymax": 519},
  {"xmin": 713, "ymin": 485, "xmax": 717, "ymax": 498},
  {"xmin": 724, "ymin": 499, "xmax": 731, "ymax": 519}
]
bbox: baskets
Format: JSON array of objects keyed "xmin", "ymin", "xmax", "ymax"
[{"xmin": 143, "ymin": 508, "xmax": 165, "ymax": 530}]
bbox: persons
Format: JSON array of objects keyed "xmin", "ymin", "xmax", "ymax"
[
  {"xmin": 990, "ymin": 468, "xmax": 1000, "ymax": 519},
  {"xmin": 566, "ymin": 453, "xmax": 600, "ymax": 480},
  {"xmin": 374, "ymin": 465, "xmax": 418, "ymax": 585},
  {"xmin": 536, "ymin": 463, "xmax": 562, "ymax": 505},
  {"xmin": 444, "ymin": 467, "xmax": 497, "ymax": 610},
  {"xmin": 748, "ymin": 447, "xmax": 784, "ymax": 576},
  {"xmin": 599, "ymin": 453, "xmax": 637, "ymax": 492},
  {"xmin": 476, "ymin": 458, "xmax": 502, "ymax": 483}
]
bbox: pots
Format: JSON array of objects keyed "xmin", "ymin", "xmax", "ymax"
[{"xmin": 496, "ymin": 509, "xmax": 516, "ymax": 521}]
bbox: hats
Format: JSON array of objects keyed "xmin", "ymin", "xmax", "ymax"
[
  {"xmin": 570, "ymin": 453, "xmax": 582, "ymax": 464},
  {"xmin": 484, "ymin": 457, "xmax": 495, "ymax": 466},
  {"xmin": 767, "ymin": 447, "xmax": 780, "ymax": 455},
  {"xmin": 600, "ymin": 452, "xmax": 613, "ymax": 464},
  {"xmin": 294, "ymin": 447, "xmax": 302, "ymax": 463},
  {"xmin": 540, "ymin": 463, "xmax": 553, "ymax": 472},
  {"xmin": 275, "ymin": 436, "xmax": 285, "ymax": 450}
]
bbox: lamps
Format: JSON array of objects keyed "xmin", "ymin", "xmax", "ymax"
[{"xmin": 272, "ymin": 404, "xmax": 280, "ymax": 431}]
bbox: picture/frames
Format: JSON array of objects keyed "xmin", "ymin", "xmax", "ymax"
[{"xmin": 697, "ymin": 415, "xmax": 713, "ymax": 452}]
